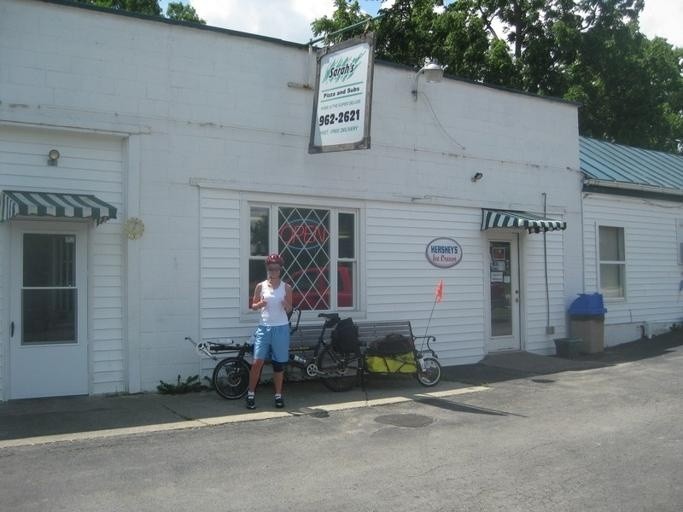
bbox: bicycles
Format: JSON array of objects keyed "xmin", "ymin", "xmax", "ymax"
[{"xmin": 212, "ymin": 312, "xmax": 364, "ymax": 399}]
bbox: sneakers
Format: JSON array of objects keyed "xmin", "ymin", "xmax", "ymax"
[
  {"xmin": 246, "ymin": 394, "xmax": 256, "ymax": 410},
  {"xmin": 275, "ymin": 395, "xmax": 284, "ymax": 408}
]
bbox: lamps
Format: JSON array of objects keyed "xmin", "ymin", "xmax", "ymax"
[
  {"xmin": 471, "ymin": 172, "xmax": 483, "ymax": 183},
  {"xmin": 46, "ymin": 149, "xmax": 60, "ymax": 166},
  {"xmin": 409, "ymin": 63, "xmax": 444, "ymax": 101}
]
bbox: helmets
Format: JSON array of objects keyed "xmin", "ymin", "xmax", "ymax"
[{"xmin": 267, "ymin": 254, "xmax": 282, "ymax": 263}]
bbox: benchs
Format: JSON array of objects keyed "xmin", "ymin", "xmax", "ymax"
[{"xmin": 287, "ymin": 319, "xmax": 415, "ymax": 387}]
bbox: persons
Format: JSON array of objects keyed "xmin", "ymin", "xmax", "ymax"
[{"xmin": 243, "ymin": 252, "xmax": 293, "ymax": 411}]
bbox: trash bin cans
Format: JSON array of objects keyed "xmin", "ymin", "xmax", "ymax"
[{"xmin": 568, "ymin": 293, "xmax": 607, "ymax": 354}]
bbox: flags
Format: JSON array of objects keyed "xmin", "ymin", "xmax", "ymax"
[{"xmin": 436, "ymin": 280, "xmax": 444, "ymax": 304}]
